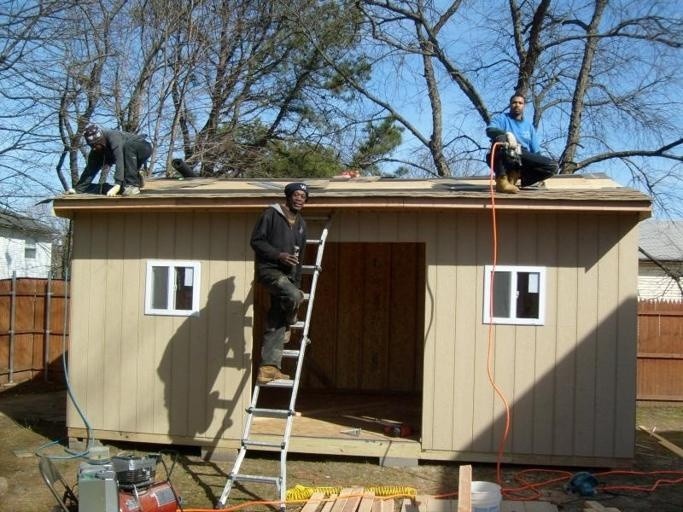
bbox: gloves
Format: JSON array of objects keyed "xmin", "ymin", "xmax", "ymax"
[
  {"xmin": 106, "ymin": 184, "xmax": 120, "ymax": 196},
  {"xmin": 64, "ymin": 188, "xmax": 75, "ymax": 194}
]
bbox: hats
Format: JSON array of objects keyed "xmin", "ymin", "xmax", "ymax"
[
  {"xmin": 82, "ymin": 124, "xmax": 106, "ymax": 152},
  {"xmin": 284, "ymin": 182, "xmax": 308, "ymax": 203}
]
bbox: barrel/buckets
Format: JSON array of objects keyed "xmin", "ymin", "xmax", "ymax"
[{"xmin": 471, "ymin": 480, "xmax": 504, "ymax": 512}]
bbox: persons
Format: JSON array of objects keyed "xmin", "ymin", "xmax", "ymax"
[
  {"xmin": 59, "ymin": 123, "xmax": 152, "ymax": 197},
  {"xmin": 247, "ymin": 182, "xmax": 309, "ymax": 383},
  {"xmin": 483, "ymin": 91, "xmax": 560, "ymax": 195}
]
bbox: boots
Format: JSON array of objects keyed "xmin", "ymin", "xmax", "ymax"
[
  {"xmin": 508, "ymin": 170, "xmax": 519, "ymax": 185},
  {"xmin": 495, "ymin": 175, "xmax": 517, "ymax": 192}
]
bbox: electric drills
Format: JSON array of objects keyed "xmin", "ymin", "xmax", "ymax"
[{"xmin": 500, "ymin": 141, "xmax": 523, "ymax": 166}]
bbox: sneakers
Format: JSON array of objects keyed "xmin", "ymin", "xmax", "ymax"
[
  {"xmin": 256, "ymin": 366, "xmax": 289, "ymax": 384},
  {"xmin": 505, "ymin": 131, "xmax": 516, "ymax": 150},
  {"xmin": 283, "ymin": 321, "xmax": 291, "ymax": 344},
  {"xmin": 121, "ymin": 185, "xmax": 140, "ymax": 196}
]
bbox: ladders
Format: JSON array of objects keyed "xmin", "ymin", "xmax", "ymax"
[{"xmin": 214, "ymin": 211, "xmax": 337, "ymax": 511}]
